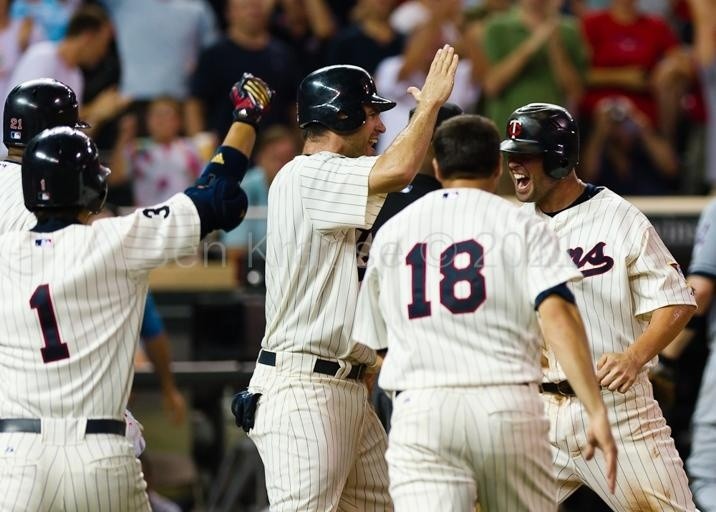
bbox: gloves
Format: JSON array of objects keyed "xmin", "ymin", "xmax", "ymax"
[
  {"xmin": 230, "ymin": 390, "xmax": 263, "ymax": 431},
  {"xmin": 229, "ymin": 72, "xmax": 275, "ymax": 131}
]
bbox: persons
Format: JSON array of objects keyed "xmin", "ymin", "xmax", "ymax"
[
  {"xmin": 2, "ymin": 72, "xmax": 271, "ymax": 511},
  {"xmin": 678, "ymin": 195, "xmax": 715, "ymax": 510},
  {"xmin": 233, "ymin": 42, "xmax": 459, "ymax": 511},
  {"xmin": 358, "ymin": 115, "xmax": 618, "ymax": 511},
  {"xmin": 0, "ymin": 0, "xmax": 715, "ymax": 249},
  {"xmin": 498, "ymin": 103, "xmax": 698, "ymax": 512}
]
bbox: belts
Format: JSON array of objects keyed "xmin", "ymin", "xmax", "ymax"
[
  {"xmin": 258, "ymin": 350, "xmax": 367, "ymax": 383},
  {"xmin": 540, "ymin": 380, "xmax": 603, "ymax": 398},
  {"xmin": 1, "ymin": 420, "xmax": 125, "ymax": 436}
]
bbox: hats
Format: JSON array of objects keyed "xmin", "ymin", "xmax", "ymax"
[{"xmin": 409, "ymin": 104, "xmax": 461, "ymax": 126}]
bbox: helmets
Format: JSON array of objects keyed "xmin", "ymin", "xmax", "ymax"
[
  {"xmin": 22, "ymin": 127, "xmax": 109, "ymax": 213},
  {"xmin": 4, "ymin": 79, "xmax": 91, "ymax": 144},
  {"xmin": 298, "ymin": 65, "xmax": 396, "ymax": 135},
  {"xmin": 500, "ymin": 102, "xmax": 580, "ymax": 178}
]
